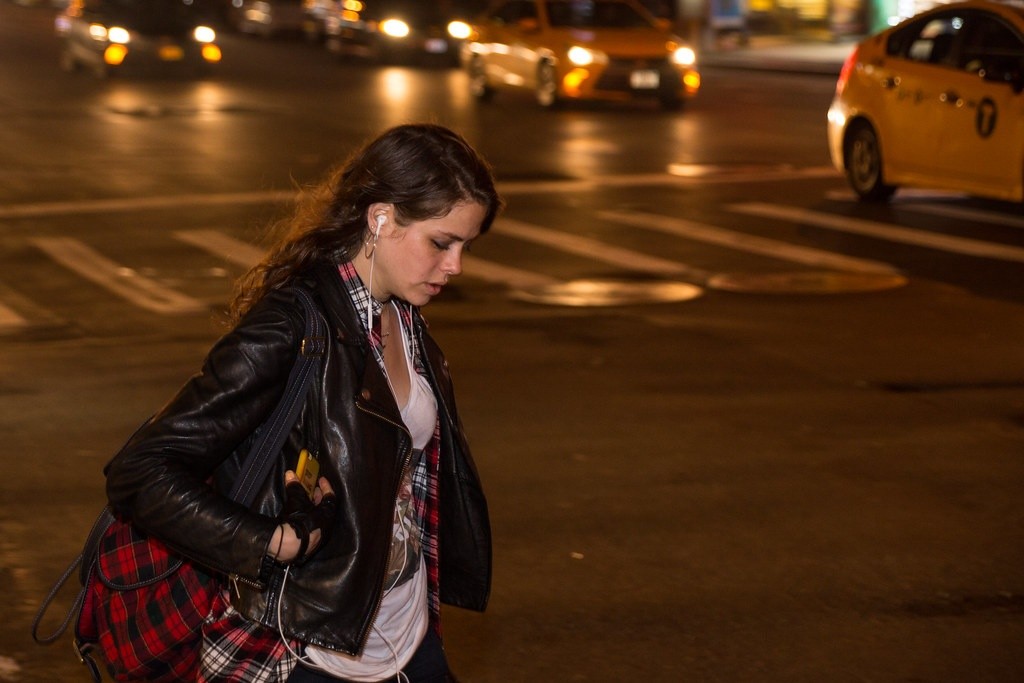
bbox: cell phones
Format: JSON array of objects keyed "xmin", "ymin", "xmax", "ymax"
[{"xmin": 296, "ymin": 449, "xmax": 319, "ymax": 500}]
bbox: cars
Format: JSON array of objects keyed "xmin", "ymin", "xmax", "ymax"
[
  {"xmin": 228, "ymin": 1, "xmax": 700, "ymax": 112},
  {"xmin": 55, "ymin": 0, "xmax": 216, "ymax": 78},
  {"xmin": 826, "ymin": 1, "xmax": 1024, "ymax": 209}
]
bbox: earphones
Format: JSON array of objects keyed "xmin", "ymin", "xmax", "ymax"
[{"xmin": 374, "ymin": 215, "xmax": 386, "ymax": 239}]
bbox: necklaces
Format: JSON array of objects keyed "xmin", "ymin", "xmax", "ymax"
[{"xmin": 381, "ymin": 305, "xmax": 392, "ymax": 337}]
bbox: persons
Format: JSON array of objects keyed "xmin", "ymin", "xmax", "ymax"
[{"xmin": 102, "ymin": 117, "xmax": 506, "ymax": 683}]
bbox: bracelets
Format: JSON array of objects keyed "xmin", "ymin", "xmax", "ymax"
[{"xmin": 272, "ymin": 523, "xmax": 284, "ymax": 560}]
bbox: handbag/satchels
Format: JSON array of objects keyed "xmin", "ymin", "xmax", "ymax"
[{"xmin": 73, "ymin": 284, "xmax": 327, "ymax": 683}]
